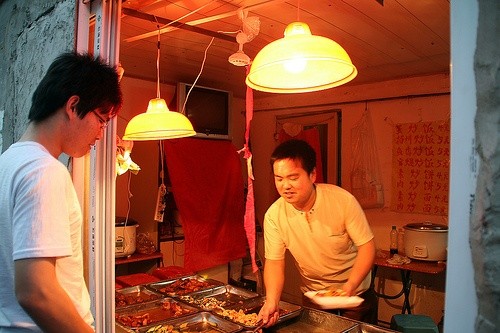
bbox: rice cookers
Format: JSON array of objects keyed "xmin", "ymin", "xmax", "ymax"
[
  {"xmin": 115, "ymin": 216, "xmax": 140, "ymax": 258},
  {"xmin": 402, "ymin": 221, "xmax": 448, "ymax": 263}
]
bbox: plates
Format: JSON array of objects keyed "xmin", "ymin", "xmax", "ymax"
[{"xmin": 304, "ymin": 290, "xmax": 365, "ymax": 309}]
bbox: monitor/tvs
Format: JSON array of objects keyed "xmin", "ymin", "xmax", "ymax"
[{"xmin": 170, "ymin": 82, "xmax": 233, "ymax": 140}]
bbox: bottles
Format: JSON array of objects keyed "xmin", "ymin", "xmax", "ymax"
[
  {"xmin": 390, "ymin": 226, "xmax": 398, "ymax": 254},
  {"xmin": 397, "ymin": 229, "xmax": 405, "ymax": 257}
]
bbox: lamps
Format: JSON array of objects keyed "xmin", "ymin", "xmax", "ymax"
[
  {"xmin": 245, "ymin": 0, "xmax": 359, "ymax": 93},
  {"xmin": 122, "ymin": 28, "xmax": 196, "ymax": 141}
]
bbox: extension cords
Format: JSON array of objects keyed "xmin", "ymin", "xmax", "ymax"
[{"xmin": 154, "ymin": 186, "xmax": 168, "ymax": 222}]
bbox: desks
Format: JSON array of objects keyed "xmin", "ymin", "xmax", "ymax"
[
  {"xmin": 369, "ymin": 249, "xmax": 446, "ymax": 313},
  {"xmin": 114, "ymin": 253, "xmax": 162, "ymax": 268}
]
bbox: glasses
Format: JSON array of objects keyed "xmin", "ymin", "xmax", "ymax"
[{"xmin": 93, "ymin": 111, "xmax": 110, "ymax": 129}]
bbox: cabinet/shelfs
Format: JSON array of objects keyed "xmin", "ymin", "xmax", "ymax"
[{"xmin": 130, "ymin": 142, "xmax": 263, "ymax": 285}]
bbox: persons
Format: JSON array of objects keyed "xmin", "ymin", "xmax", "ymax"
[
  {"xmin": 256, "ymin": 139, "xmax": 377, "ymax": 333},
  {"xmin": 0, "ymin": 51, "xmax": 123, "ymax": 333}
]
